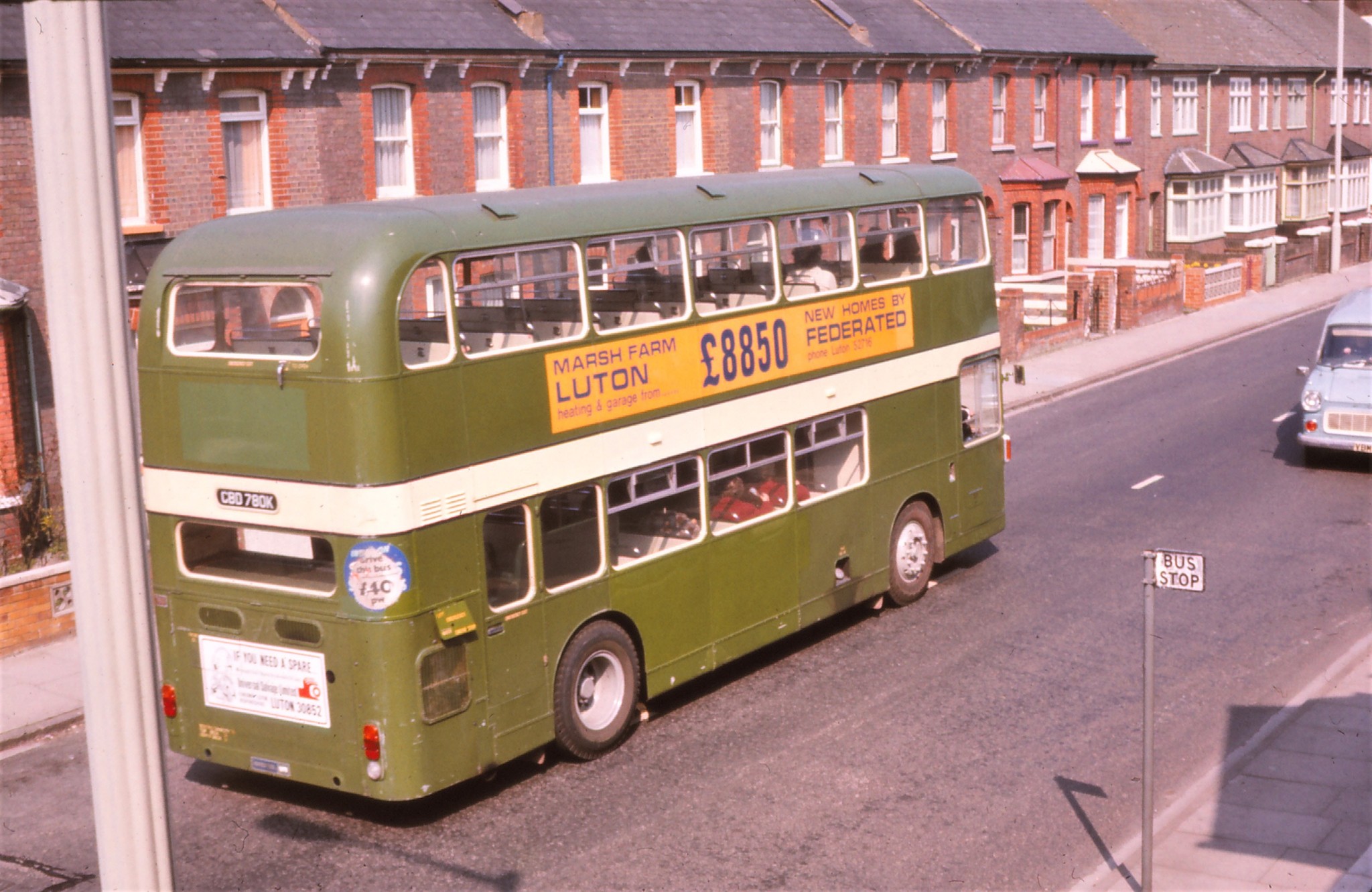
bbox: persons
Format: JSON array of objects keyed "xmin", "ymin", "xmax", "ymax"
[
  {"xmin": 711, "ymin": 475, "xmax": 773, "ymax": 526},
  {"xmin": 888, "ymin": 230, "xmax": 922, "ymax": 263},
  {"xmin": 783, "ymin": 244, "xmax": 837, "ymax": 297},
  {"xmin": 752, "ymin": 457, "xmax": 810, "ymax": 507},
  {"xmin": 858, "ymin": 227, "xmax": 887, "ymax": 263}
]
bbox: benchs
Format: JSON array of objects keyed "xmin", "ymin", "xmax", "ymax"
[
  {"xmin": 230, "ymin": 262, "xmax": 941, "ymax": 365},
  {"xmin": 484, "ymin": 473, "xmax": 825, "ymax": 608}
]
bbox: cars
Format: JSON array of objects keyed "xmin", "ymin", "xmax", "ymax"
[{"xmin": 1296, "ymin": 290, "xmax": 1371, "ymax": 453}]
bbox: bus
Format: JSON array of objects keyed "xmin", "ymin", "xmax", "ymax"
[{"xmin": 134, "ymin": 166, "xmax": 1016, "ymax": 789}]
